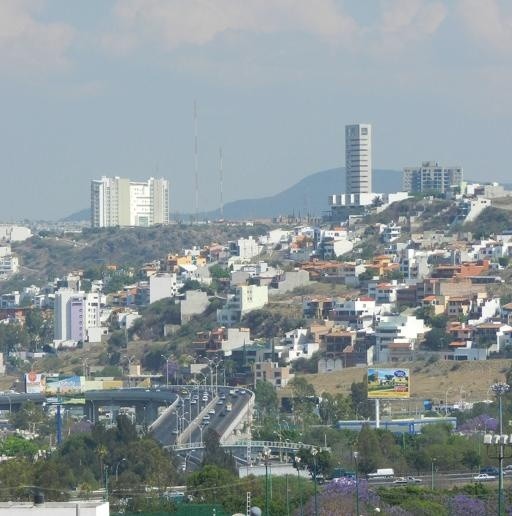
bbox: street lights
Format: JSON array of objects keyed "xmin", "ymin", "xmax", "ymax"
[
  {"xmin": 78, "ymin": 352, "xmax": 225, "ymax": 447},
  {"xmin": 257, "ymin": 446, "xmax": 275, "ymax": 514},
  {"xmin": 353, "ymin": 451, "xmax": 362, "ymax": 516},
  {"xmin": 311, "ymin": 448, "xmax": 322, "ymax": 515},
  {"xmin": 429, "ymin": 456, "xmax": 437, "ymax": 490},
  {"xmin": 293, "ymin": 455, "xmax": 305, "ymax": 515},
  {"xmin": 490, "ymin": 383, "xmax": 508, "ymax": 514}
]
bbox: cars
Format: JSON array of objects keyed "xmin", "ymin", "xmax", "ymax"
[
  {"xmin": 473, "ymin": 474, "xmax": 496, "ymax": 481},
  {"xmin": 168, "ymin": 383, "xmax": 248, "ymax": 435}
]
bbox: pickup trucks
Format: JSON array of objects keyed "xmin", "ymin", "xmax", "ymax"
[{"xmin": 396, "ymin": 475, "xmax": 422, "ymax": 485}]
